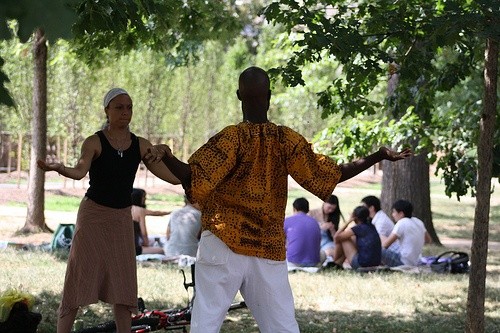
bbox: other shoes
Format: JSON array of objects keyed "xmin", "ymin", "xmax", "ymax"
[{"xmin": 325, "ymin": 261, "xmax": 345, "ymax": 270}]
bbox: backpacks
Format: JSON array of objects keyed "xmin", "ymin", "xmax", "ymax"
[{"xmin": 430, "ymin": 250, "xmax": 469, "ymax": 274}]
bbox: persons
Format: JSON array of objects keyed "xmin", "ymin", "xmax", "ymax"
[
  {"xmin": 283, "ymin": 197, "xmax": 330, "ymax": 267},
  {"xmin": 163, "ymin": 194, "xmax": 202, "ymax": 257},
  {"xmin": 37, "ymin": 88, "xmax": 182, "ymax": 333},
  {"xmin": 306, "ymin": 194, "xmax": 339, "ymax": 249},
  {"xmin": 328, "ymin": 206, "xmax": 381, "ymax": 270},
  {"xmin": 131, "ymin": 188, "xmax": 172, "ymax": 255},
  {"xmin": 361, "ymin": 196, "xmax": 399, "ymax": 251},
  {"xmin": 145, "ymin": 66, "xmax": 412, "ymax": 333},
  {"xmin": 380, "ymin": 200, "xmax": 432, "ymax": 267}
]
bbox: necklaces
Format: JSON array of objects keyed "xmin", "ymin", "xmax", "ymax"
[{"xmin": 105, "ymin": 128, "xmax": 128, "ymax": 157}]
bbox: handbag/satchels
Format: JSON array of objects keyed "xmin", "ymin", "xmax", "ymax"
[{"xmin": 51, "ymin": 223, "xmax": 75, "ymax": 253}]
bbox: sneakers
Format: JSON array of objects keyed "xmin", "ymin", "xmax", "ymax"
[{"xmin": 322, "ymin": 256, "xmax": 334, "ymax": 267}]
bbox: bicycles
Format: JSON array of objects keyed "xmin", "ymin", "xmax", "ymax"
[{"xmin": 73, "ymin": 262, "xmax": 247, "ymax": 333}]
